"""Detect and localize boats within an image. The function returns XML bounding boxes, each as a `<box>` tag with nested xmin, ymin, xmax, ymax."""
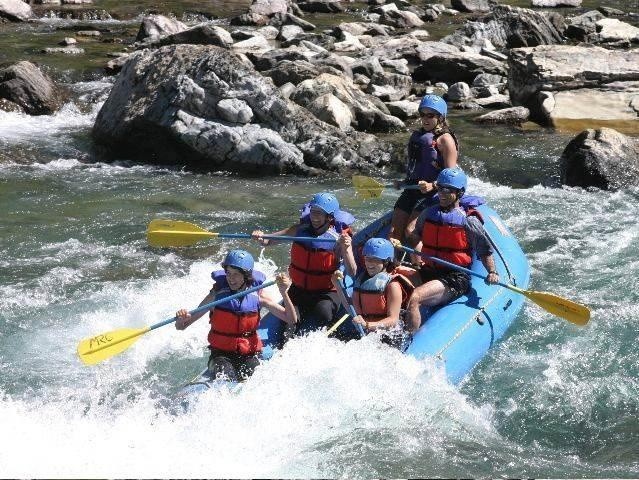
<box><xmin>170</xmin><ymin>193</ymin><xmax>531</xmax><ymax>415</ymax></box>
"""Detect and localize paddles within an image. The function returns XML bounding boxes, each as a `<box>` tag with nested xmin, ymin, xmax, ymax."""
<box><xmin>393</xmin><ymin>243</ymin><xmax>590</xmax><ymax>326</ymax></box>
<box><xmin>353</xmin><ymin>175</ymin><xmax>422</xmax><ymax>200</ymax></box>
<box><xmin>77</xmin><ymin>277</ymin><xmax>277</xmax><ymax>367</ymax></box>
<box><xmin>147</xmin><ymin>219</ymin><xmax>339</xmax><ymax>248</ymax></box>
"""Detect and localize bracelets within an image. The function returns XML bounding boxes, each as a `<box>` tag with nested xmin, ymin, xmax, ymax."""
<box><xmin>432</xmin><ymin>180</ymin><xmax>436</xmax><ymax>191</ymax></box>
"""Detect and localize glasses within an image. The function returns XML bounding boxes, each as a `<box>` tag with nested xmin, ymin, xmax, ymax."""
<box><xmin>437</xmin><ymin>185</ymin><xmax>455</xmax><ymax>194</ymax></box>
<box><xmin>420</xmin><ymin>111</ymin><xmax>440</xmax><ymax>118</ymax></box>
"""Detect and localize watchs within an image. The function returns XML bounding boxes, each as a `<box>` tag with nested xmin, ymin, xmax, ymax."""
<box><xmin>489</xmin><ymin>270</ymin><xmax>499</xmax><ymax>275</ymax></box>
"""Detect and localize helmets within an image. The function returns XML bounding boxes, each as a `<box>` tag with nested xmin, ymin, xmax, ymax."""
<box><xmin>362</xmin><ymin>236</ymin><xmax>395</xmax><ymax>260</ymax></box>
<box><xmin>309</xmin><ymin>192</ymin><xmax>340</xmax><ymax>218</ymax></box>
<box><xmin>224</xmin><ymin>249</ymin><xmax>254</xmax><ymax>273</ymax></box>
<box><xmin>418</xmin><ymin>94</ymin><xmax>448</xmax><ymax>117</ymax></box>
<box><xmin>436</xmin><ymin>167</ymin><xmax>467</xmax><ymax>191</ymax></box>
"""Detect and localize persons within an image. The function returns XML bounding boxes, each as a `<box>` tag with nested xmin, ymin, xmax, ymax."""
<box><xmin>388</xmin><ymin>94</ymin><xmax>459</xmax><ymax>268</ymax></box>
<box><xmin>390</xmin><ymin>167</ymin><xmax>499</xmax><ymax>336</ymax></box>
<box><xmin>330</xmin><ymin>238</ymin><xmax>416</xmax><ymax>354</ymax></box>
<box><xmin>252</xmin><ymin>192</ymin><xmax>357</xmax><ymax>348</ymax></box>
<box><xmin>175</xmin><ymin>249</ymin><xmax>298</xmax><ymax>383</ymax></box>
